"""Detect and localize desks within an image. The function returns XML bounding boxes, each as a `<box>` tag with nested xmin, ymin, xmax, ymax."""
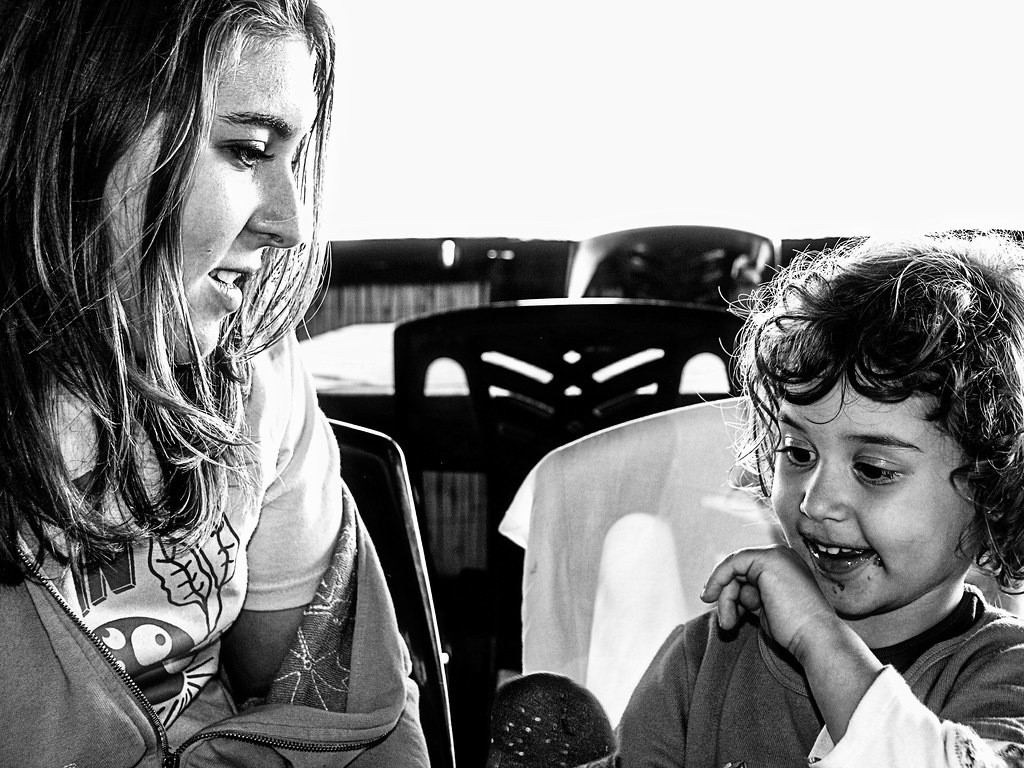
<box><xmin>299</xmin><ymin>322</ymin><xmax>752</xmax><ymax>474</ymax></box>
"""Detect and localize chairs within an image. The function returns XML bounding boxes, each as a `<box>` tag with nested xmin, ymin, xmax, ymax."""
<box><xmin>294</xmin><ymin>227</ymin><xmax>869</xmax><ymax>768</ymax></box>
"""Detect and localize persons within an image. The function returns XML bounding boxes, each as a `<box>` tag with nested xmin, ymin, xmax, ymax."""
<box><xmin>2</xmin><ymin>0</ymin><xmax>435</xmax><ymax>768</ymax></box>
<box><xmin>614</xmin><ymin>227</ymin><xmax>1024</xmax><ymax>768</ymax></box>
